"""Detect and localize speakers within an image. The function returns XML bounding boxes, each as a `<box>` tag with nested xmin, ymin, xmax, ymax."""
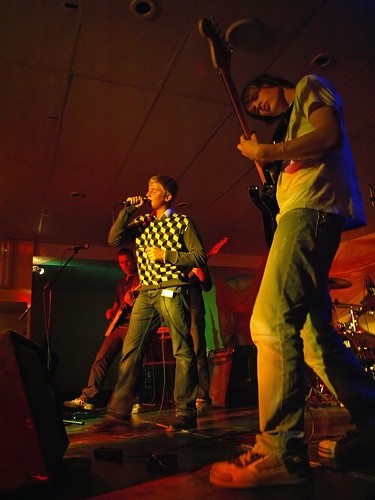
<box><xmin>207</xmin><ymin>345</ymin><xmax>259</xmax><ymax>409</ymax></box>
<box><xmin>0</xmin><ymin>330</ymin><xmax>70</xmax><ymax>500</ymax></box>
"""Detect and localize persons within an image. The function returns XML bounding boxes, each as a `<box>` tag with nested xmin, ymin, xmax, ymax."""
<box><xmin>60</xmin><ymin>175</ymin><xmax>212</xmax><ymax>432</ymax></box>
<box><xmin>208</xmin><ymin>74</ymin><xmax>375</xmax><ymax>490</ymax></box>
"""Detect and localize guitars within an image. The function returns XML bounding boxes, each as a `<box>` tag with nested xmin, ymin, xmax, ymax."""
<box><xmin>188</xmin><ymin>237</ymin><xmax>228</xmax><ymax>278</ymax></box>
<box><xmin>104</xmin><ymin>283</ymin><xmax>142</xmax><ymax>337</ymax></box>
<box><xmin>199</xmin><ymin>15</ymin><xmax>279</xmax><ymax>250</ymax></box>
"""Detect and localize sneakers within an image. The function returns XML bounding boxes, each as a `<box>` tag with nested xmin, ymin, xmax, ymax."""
<box><xmin>63</xmin><ymin>398</ymin><xmax>94</xmax><ymax>410</ymax></box>
<box><xmin>318</xmin><ymin>441</ymin><xmax>338</xmax><ymax>459</ymax></box>
<box><xmin>132</xmin><ymin>404</ymin><xmax>144</xmax><ymax>414</ymax></box>
<box><xmin>209</xmin><ymin>444</ymin><xmax>305</xmax><ymax>486</ymax></box>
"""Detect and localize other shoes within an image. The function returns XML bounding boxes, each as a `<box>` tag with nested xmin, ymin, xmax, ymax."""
<box><xmin>88</xmin><ymin>415</ymin><xmax>126</xmax><ymax>433</ymax></box>
<box><xmin>194</xmin><ymin>400</ymin><xmax>213</xmax><ymax>417</ymax></box>
<box><xmin>172</xmin><ymin>410</ymin><xmax>196</xmax><ymax>430</ymax></box>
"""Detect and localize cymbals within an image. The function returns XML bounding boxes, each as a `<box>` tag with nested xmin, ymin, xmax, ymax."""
<box><xmin>327</xmin><ymin>276</ymin><xmax>352</xmax><ymax>290</ymax></box>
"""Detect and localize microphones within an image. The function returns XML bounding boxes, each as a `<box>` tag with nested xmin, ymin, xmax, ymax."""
<box><xmin>120</xmin><ymin>197</ymin><xmax>147</xmax><ymax>205</ymax></box>
<box><xmin>67</xmin><ymin>243</ymin><xmax>89</xmax><ymax>250</ymax></box>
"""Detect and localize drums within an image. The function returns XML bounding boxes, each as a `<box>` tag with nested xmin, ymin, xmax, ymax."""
<box><xmin>339</xmin><ymin>328</ymin><xmax>375</xmax><ymax>377</ymax></box>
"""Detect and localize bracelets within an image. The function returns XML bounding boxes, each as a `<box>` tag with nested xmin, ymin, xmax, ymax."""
<box><xmin>280</xmin><ymin>142</ymin><xmax>288</xmax><ymax>161</ymax></box>
